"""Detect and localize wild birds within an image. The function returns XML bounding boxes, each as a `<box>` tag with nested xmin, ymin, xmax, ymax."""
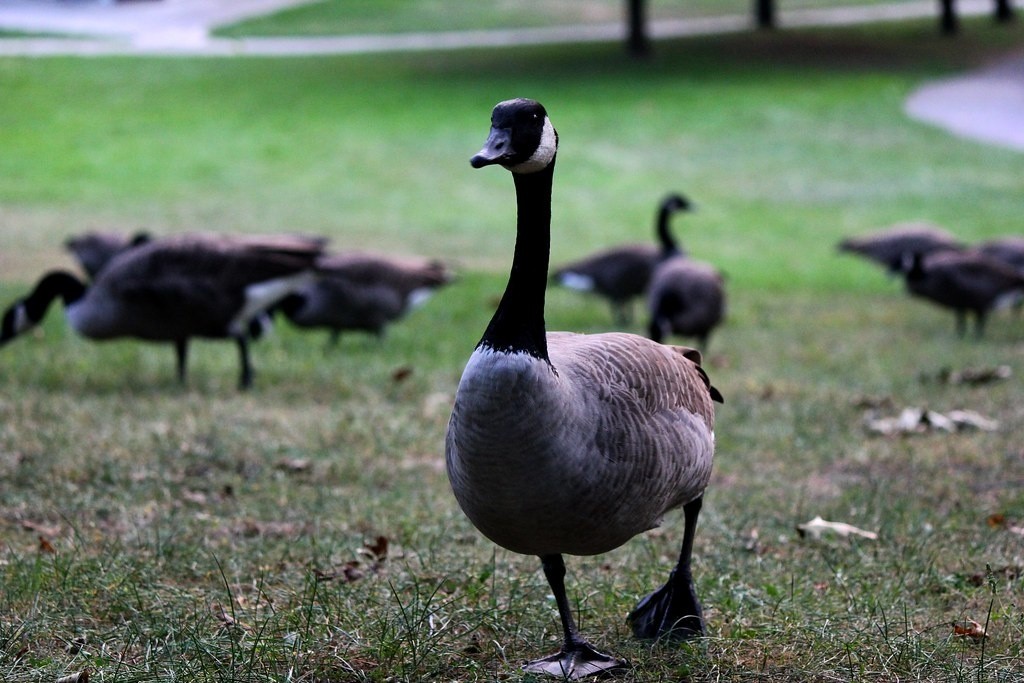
<box><xmin>0</xmin><ymin>225</ymin><xmax>458</xmax><ymax>391</ymax></box>
<box><xmin>445</xmin><ymin>96</ymin><xmax>724</xmax><ymax>683</ymax></box>
<box><xmin>837</xmin><ymin>223</ymin><xmax>1024</xmax><ymax>341</ymax></box>
<box><xmin>549</xmin><ymin>190</ymin><xmax>725</xmax><ymax>356</ymax></box>
